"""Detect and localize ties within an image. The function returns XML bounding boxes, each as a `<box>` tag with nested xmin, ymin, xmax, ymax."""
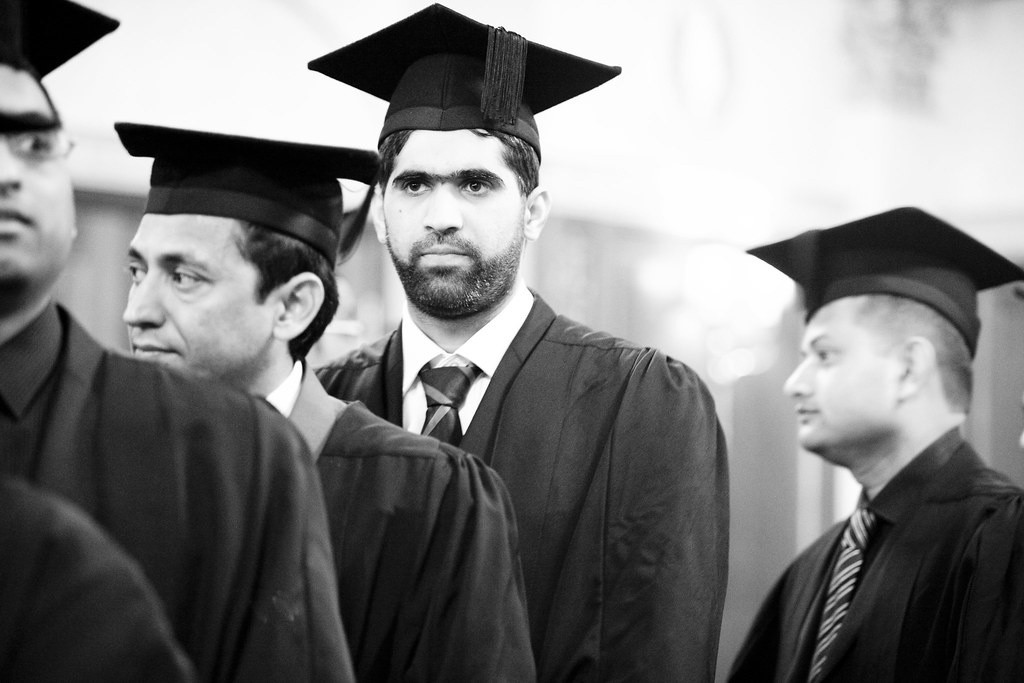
<box><xmin>807</xmin><ymin>510</ymin><xmax>875</xmax><ymax>683</ymax></box>
<box><xmin>415</xmin><ymin>362</ymin><xmax>482</xmax><ymax>447</ymax></box>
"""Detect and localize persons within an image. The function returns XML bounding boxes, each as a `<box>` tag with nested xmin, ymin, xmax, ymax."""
<box><xmin>114</xmin><ymin>122</ymin><xmax>540</xmax><ymax>683</ymax></box>
<box><xmin>307</xmin><ymin>2</ymin><xmax>733</xmax><ymax>683</ymax></box>
<box><xmin>1</xmin><ymin>0</ymin><xmax>356</xmax><ymax>683</ymax></box>
<box><xmin>726</xmin><ymin>202</ymin><xmax>1024</xmax><ymax>682</ymax></box>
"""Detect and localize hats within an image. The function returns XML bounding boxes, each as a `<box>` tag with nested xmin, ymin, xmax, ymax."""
<box><xmin>308</xmin><ymin>3</ymin><xmax>622</xmax><ymax>167</ymax></box>
<box><xmin>745</xmin><ymin>206</ymin><xmax>1024</xmax><ymax>361</ymax></box>
<box><xmin>0</xmin><ymin>0</ymin><xmax>120</xmax><ymax>82</ymax></box>
<box><xmin>114</xmin><ymin>121</ymin><xmax>382</xmax><ymax>271</ymax></box>
